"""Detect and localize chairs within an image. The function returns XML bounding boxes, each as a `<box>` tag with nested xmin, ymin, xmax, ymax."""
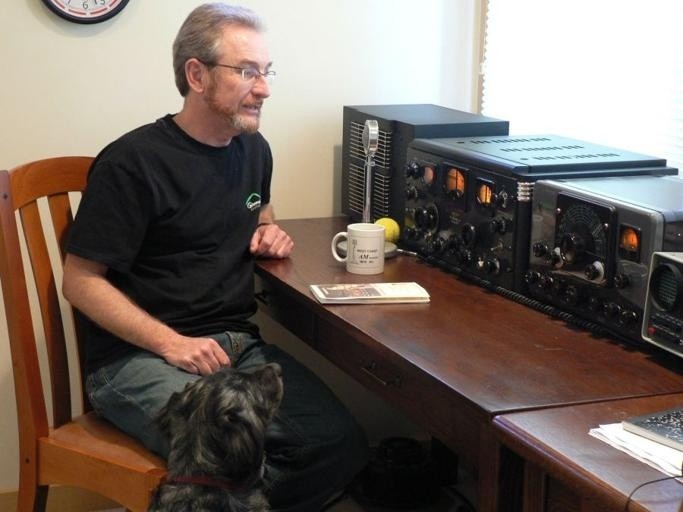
<box><xmin>0</xmin><ymin>156</ymin><xmax>167</xmax><ymax>512</ymax></box>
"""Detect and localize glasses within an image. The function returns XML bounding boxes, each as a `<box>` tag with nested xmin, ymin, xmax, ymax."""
<box><xmin>215</xmin><ymin>61</ymin><xmax>278</xmax><ymax>85</ymax></box>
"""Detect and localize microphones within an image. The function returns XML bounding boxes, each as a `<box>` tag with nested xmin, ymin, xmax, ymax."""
<box><xmin>337</xmin><ymin>119</ymin><xmax>398</xmax><ymax>260</ymax></box>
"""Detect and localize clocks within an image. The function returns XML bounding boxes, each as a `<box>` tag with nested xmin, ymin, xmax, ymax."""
<box><xmin>42</xmin><ymin>1</ymin><xmax>132</xmax><ymax>24</ymax></box>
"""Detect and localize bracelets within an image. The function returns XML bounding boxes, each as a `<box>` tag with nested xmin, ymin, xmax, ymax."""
<box><xmin>254</xmin><ymin>221</ymin><xmax>272</xmax><ymax>228</ymax></box>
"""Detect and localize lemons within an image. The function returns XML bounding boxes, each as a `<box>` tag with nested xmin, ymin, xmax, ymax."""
<box><xmin>374</xmin><ymin>217</ymin><xmax>401</xmax><ymax>242</ymax></box>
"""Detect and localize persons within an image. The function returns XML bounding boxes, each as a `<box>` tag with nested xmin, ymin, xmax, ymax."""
<box><xmin>55</xmin><ymin>1</ymin><xmax>371</xmax><ymax>512</ymax></box>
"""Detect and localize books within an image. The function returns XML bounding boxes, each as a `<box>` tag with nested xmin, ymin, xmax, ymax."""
<box><xmin>308</xmin><ymin>280</ymin><xmax>433</xmax><ymax>306</ymax></box>
<box><xmin>588</xmin><ymin>408</ymin><xmax>683</xmax><ymax>485</ymax></box>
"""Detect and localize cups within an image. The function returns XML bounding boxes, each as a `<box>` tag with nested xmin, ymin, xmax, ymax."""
<box><xmin>330</xmin><ymin>222</ymin><xmax>386</xmax><ymax>276</ymax></box>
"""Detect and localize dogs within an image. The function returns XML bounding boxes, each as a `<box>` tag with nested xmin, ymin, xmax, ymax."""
<box><xmin>146</xmin><ymin>361</ymin><xmax>287</xmax><ymax>512</ymax></box>
